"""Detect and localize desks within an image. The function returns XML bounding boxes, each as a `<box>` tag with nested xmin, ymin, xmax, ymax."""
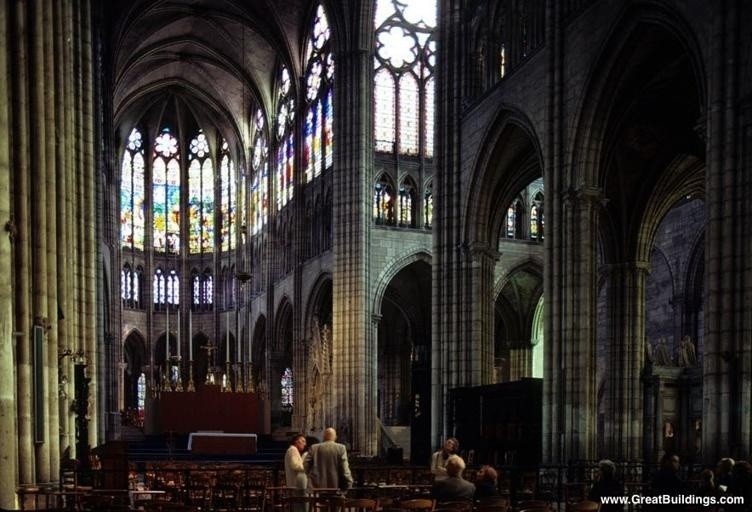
<box><xmin>187</xmin><ymin>432</ymin><xmax>258</xmax><ymax>454</ymax></box>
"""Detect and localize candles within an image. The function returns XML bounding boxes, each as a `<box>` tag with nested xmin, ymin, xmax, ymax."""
<box><xmin>223</xmin><ymin>374</ymin><xmax>226</xmax><ymax>387</ymax></box>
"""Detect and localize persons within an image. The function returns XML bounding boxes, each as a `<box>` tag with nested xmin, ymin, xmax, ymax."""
<box><xmin>308</xmin><ymin>426</ymin><xmax>353</xmax><ymax>511</ymax></box>
<box><xmin>282</xmin><ymin>434</ymin><xmax>310</xmax><ymax>511</ymax></box>
<box><xmin>475</xmin><ymin>465</ymin><xmax>498</xmax><ymax>496</ymax></box>
<box><xmin>430</xmin><ymin>437</ymin><xmax>460</xmax><ymax>482</ymax></box>
<box><xmin>429</xmin><ymin>454</ymin><xmax>475</xmax><ymax>502</ymax></box>
<box><xmin>649</xmin><ymin>452</ymin><xmax>752</xmax><ymax>512</ymax></box>
<box><xmin>586</xmin><ymin>458</ymin><xmax>623</xmax><ymax>512</ymax></box>
